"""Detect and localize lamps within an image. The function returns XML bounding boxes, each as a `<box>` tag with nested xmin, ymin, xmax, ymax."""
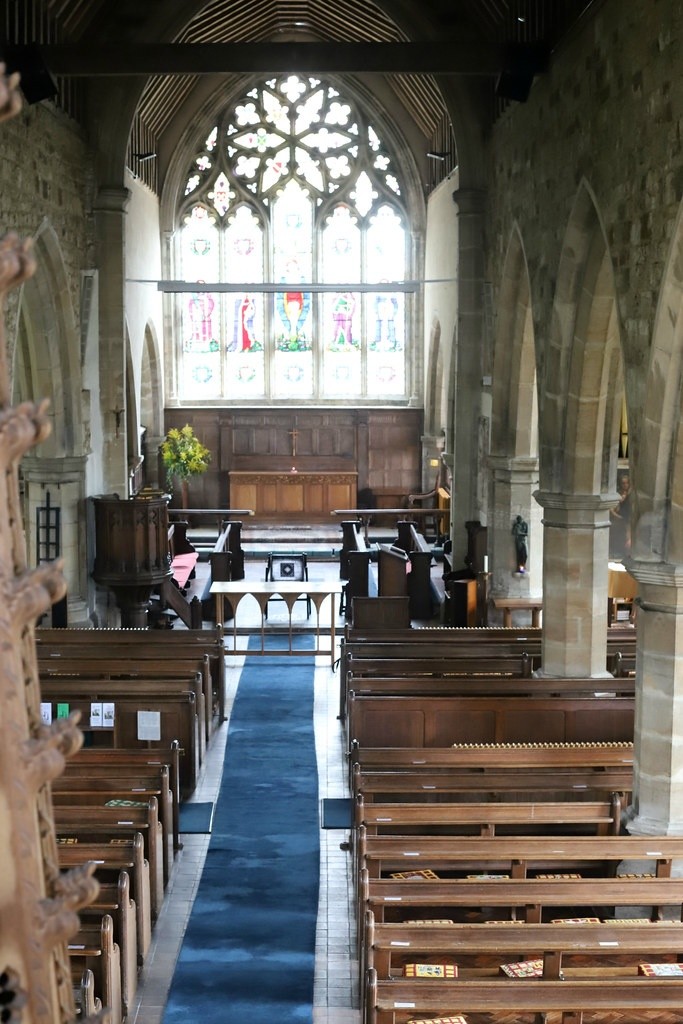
<box><xmin>132</xmin><ymin>153</ymin><xmax>157</xmax><ymax>162</ymax></box>
<box><xmin>427</xmin><ymin>152</ymin><xmax>455</xmax><ymax>160</ymax></box>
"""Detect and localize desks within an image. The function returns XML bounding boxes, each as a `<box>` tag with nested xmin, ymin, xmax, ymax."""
<box><xmin>371</xmin><ymin>489</ymin><xmax>410</xmax><ymax>526</ymax></box>
<box><xmin>492</xmin><ymin>598</ymin><xmax>542</xmax><ymax>628</ymax></box>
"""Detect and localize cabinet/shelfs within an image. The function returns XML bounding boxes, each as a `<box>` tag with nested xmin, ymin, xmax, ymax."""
<box><xmin>227</xmin><ymin>470</ymin><xmax>357</xmax><ymax>523</ymax></box>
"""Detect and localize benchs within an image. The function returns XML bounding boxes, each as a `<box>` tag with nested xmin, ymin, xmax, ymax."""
<box><xmin>33</xmin><ymin>622</ymin><xmax>682</xmax><ymax>1024</ymax></box>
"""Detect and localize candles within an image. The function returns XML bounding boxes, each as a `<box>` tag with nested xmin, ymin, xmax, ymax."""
<box><xmin>484</xmin><ymin>556</ymin><xmax>488</xmax><ymax>573</ymax></box>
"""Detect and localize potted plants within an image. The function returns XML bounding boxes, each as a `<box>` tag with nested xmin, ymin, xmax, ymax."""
<box><xmin>158</xmin><ymin>424</ymin><xmax>212</xmax><ymax>519</ymax></box>
